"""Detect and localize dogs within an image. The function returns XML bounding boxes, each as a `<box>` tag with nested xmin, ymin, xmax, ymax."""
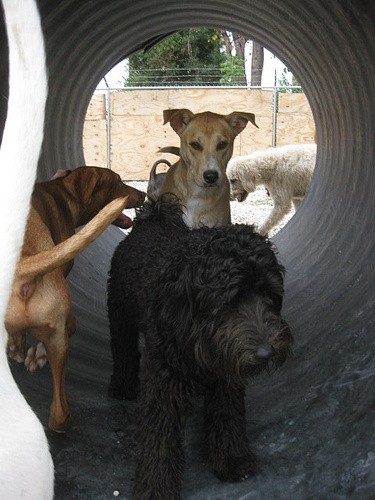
<box><xmin>225</xmin><ymin>143</ymin><xmax>319</xmax><ymax>240</ymax></box>
<box><xmin>106</xmin><ymin>191</ymin><xmax>297</xmax><ymax>500</ymax></box>
<box><xmin>3</xmin><ymin>166</ymin><xmax>147</xmax><ymax>435</ymax></box>
<box><xmin>146</xmin><ymin>108</ymin><xmax>261</xmax><ymax>231</ymax></box>
